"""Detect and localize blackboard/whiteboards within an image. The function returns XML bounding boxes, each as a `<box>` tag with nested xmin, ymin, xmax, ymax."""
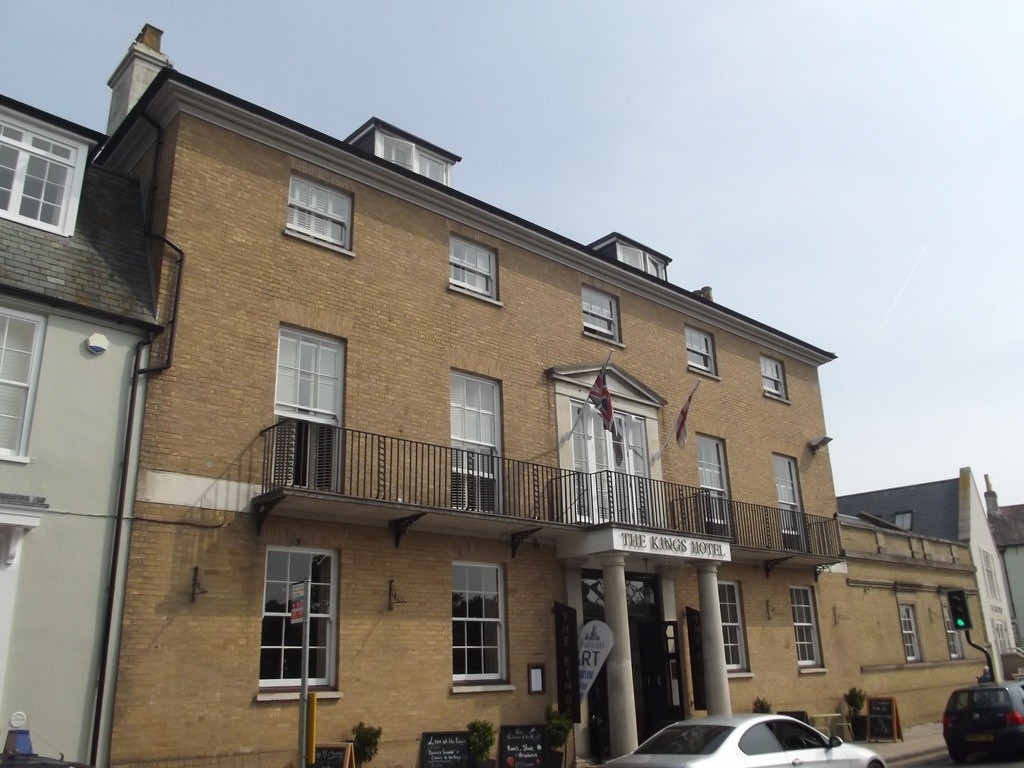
<box><xmin>314</xmin><ymin>742</ymin><xmax>349</xmax><ymax>768</ymax></box>
<box><xmin>500</xmin><ymin>724</ymin><xmax>544</xmax><ymax>768</ymax></box>
<box><xmin>777</xmin><ymin>711</ymin><xmax>805</xmax><ymax>724</ymax></box>
<box><xmin>420</xmin><ymin>730</ymin><xmax>470</xmax><ymax>768</ymax></box>
<box><xmin>866</xmin><ymin>696</ymin><xmax>896</xmax><ymax>740</ymax></box>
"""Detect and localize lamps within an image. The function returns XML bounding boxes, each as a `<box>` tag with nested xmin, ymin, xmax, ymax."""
<box><xmin>809</xmin><ymin>435</ymin><xmax>832</xmax><ymax>449</ymax></box>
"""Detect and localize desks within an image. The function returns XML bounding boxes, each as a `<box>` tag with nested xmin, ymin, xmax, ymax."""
<box><xmin>812</xmin><ymin>713</ymin><xmax>842</xmax><ymax>729</ymax></box>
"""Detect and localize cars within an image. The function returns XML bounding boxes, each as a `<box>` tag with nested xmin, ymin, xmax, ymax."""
<box><xmin>591</xmin><ymin>705</ymin><xmax>891</xmax><ymax>768</ymax></box>
<box><xmin>941</xmin><ymin>679</ymin><xmax>1024</xmax><ymax>762</ymax></box>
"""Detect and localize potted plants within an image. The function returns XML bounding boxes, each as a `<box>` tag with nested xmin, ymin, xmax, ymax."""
<box><xmin>844</xmin><ymin>687</ymin><xmax>866</xmax><ymax>741</ymax></box>
<box><xmin>467</xmin><ymin>719</ymin><xmax>499</xmax><ymax>768</ymax></box>
<box><xmin>539</xmin><ymin>706</ymin><xmax>573</xmax><ymax>768</ymax></box>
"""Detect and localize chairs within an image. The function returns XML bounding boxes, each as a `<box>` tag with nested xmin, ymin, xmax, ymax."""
<box><xmin>833</xmin><ymin>704</ymin><xmax>853</xmax><ymax>743</ymax></box>
<box><xmin>814</xmin><ymin>726</ymin><xmax>831</xmax><ymax>738</ymax></box>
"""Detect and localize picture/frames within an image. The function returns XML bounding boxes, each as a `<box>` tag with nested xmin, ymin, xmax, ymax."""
<box><xmin>528</xmin><ymin>663</ymin><xmax>546</xmax><ymax>695</ymax></box>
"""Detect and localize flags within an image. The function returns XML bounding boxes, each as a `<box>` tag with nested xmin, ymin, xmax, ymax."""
<box><xmin>587</xmin><ymin>364</ymin><xmax>618</xmax><ymax>437</ymax></box>
<box><xmin>675</xmin><ymin>395</ymin><xmax>688</xmax><ymax>450</ymax></box>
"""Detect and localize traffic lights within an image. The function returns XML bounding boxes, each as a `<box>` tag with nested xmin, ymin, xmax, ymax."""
<box><xmin>946</xmin><ymin>589</ymin><xmax>974</xmax><ymax>630</ymax></box>
